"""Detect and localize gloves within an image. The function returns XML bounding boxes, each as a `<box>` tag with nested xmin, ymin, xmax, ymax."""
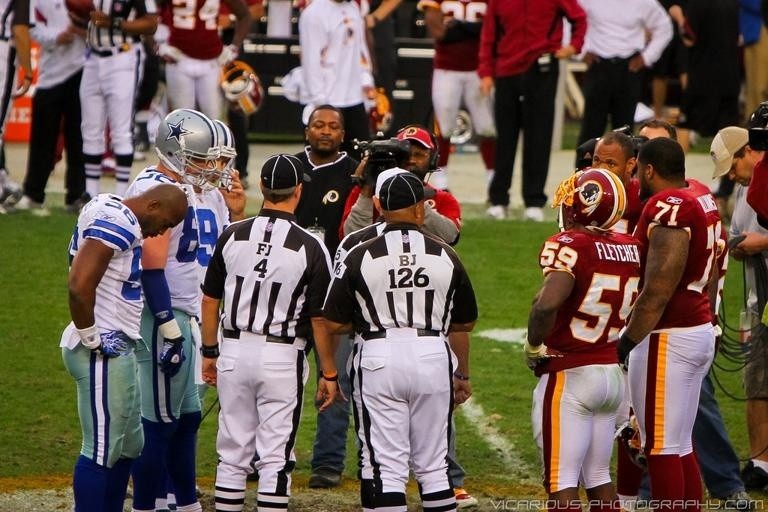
<box><xmin>96</xmin><ymin>330</ymin><xmax>128</xmax><ymax>360</ymax></box>
<box><xmin>217</xmin><ymin>44</ymin><xmax>239</xmax><ymax>62</ymax></box>
<box><xmin>523</xmin><ymin>337</ymin><xmax>548</xmax><ymax>370</ymax></box>
<box><xmin>157</xmin><ymin>335</ymin><xmax>187</xmax><ymax>378</ymax></box>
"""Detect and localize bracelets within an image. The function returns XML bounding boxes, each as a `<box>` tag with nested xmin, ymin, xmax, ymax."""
<box><xmin>322</xmin><ymin>372</ymin><xmax>339</xmax><ymax>384</ymax></box>
<box><xmin>75</xmin><ymin>326</ymin><xmax>102</xmax><ymax>350</ymax></box>
<box><xmin>199</xmin><ymin>343</ymin><xmax>218</xmax><ymax>360</ymax></box>
<box><xmin>453</xmin><ymin>373</ymin><xmax>469</xmax><ymax>382</ymax></box>
<box><xmin>525</xmin><ymin>337</ymin><xmax>542</xmax><ymax>359</ymax></box>
<box><xmin>159</xmin><ymin>319</ymin><xmax>184</xmax><ymax>342</ymax></box>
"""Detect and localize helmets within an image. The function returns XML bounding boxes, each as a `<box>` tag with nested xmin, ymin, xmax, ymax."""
<box><xmin>551</xmin><ymin>167</ymin><xmax>628</xmax><ymax>234</ymax></box>
<box><xmin>366</xmin><ymin>86</ymin><xmax>394</xmax><ymax>134</ymax></box>
<box><xmin>219</xmin><ymin>61</ymin><xmax>263</xmax><ymax>117</ymax></box>
<box><xmin>154</xmin><ymin>109</ymin><xmax>220</xmax><ymax>189</ymax></box>
<box><xmin>210</xmin><ymin>120</ymin><xmax>237</xmax><ymax>191</ymax></box>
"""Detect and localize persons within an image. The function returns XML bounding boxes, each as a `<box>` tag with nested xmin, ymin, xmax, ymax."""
<box><xmin>524</xmin><ymin>167</ymin><xmax>642</xmax><ymax>511</ymax></box>
<box><xmin>123</xmin><ymin>108</ymin><xmax>222</xmax><ymax>512</ymax></box>
<box><xmin>194</xmin><ymin>117</ymin><xmax>246</xmax><ymax>396</ymax></box>
<box><xmin>339</xmin><ymin>124</ymin><xmax>479</xmax><ymax>512</ymax></box>
<box><xmin>595</xmin><ymin>122</ymin><xmax>768</xmax><ymax>512</ymax></box>
<box><xmin>657</xmin><ymin>1</ymin><xmax>764</xmax><ymax>131</ymax></box>
<box><xmin>201</xmin><ymin>151</ymin><xmax>347</xmax><ymax>510</ymax></box>
<box><xmin>59</xmin><ymin>185</ymin><xmax>189</xmax><ymax>511</ymax></box>
<box><xmin>244</xmin><ymin>106</ymin><xmax>359</xmax><ymax>487</ymax></box>
<box><xmin>0</xmin><ymin>0</ymin><xmax>657</xmax><ymax>223</ymax></box>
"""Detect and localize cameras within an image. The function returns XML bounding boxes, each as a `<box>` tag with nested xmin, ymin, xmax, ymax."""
<box><xmin>748</xmin><ymin>102</ymin><xmax>768</xmax><ymax>151</ymax></box>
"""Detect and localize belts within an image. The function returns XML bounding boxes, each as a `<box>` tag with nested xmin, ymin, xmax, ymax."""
<box><xmin>361</xmin><ymin>328</ymin><xmax>439</xmax><ymax>339</ymax></box>
<box><xmin>222</xmin><ymin>330</ymin><xmax>294</xmax><ymax>343</ymax></box>
<box><xmin>91</xmin><ymin>47</ymin><xmax>123</xmax><ymax>58</ymax></box>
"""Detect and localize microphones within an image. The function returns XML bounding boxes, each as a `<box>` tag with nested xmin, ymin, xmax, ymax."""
<box><xmin>410</xmin><ymin>168</ymin><xmax>431</xmax><ymax>186</ymax></box>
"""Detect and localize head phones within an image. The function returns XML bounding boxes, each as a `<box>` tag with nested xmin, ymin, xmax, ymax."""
<box><xmin>394</xmin><ymin>120</ymin><xmax>440</xmax><ymax>169</ymax></box>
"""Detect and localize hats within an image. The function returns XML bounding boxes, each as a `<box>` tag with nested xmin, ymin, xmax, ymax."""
<box><xmin>375</xmin><ymin>166</ymin><xmax>410</xmax><ymax>199</ymax></box>
<box><xmin>745</xmin><ymin>102</ymin><xmax>768</xmax><ymax>131</ymax></box>
<box><xmin>397</xmin><ymin>127</ymin><xmax>434</xmax><ymax>149</ymax></box>
<box><xmin>709</xmin><ymin>125</ymin><xmax>750</xmax><ymax>180</ymax></box>
<box><xmin>379</xmin><ymin>172</ymin><xmax>435</xmax><ymax>210</ymax></box>
<box><xmin>260</xmin><ymin>153</ymin><xmax>311</xmax><ymax>189</ymax></box>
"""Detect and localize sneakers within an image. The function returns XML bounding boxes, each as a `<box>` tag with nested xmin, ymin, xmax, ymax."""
<box><xmin>719</xmin><ymin>491</ymin><xmax>756</xmax><ymax>511</ymax></box>
<box><xmin>523</xmin><ymin>206</ymin><xmax>544</xmax><ymax>223</ymax></box>
<box><xmin>453</xmin><ymin>487</ymin><xmax>479</xmax><ymax>508</ymax></box>
<box><xmin>485</xmin><ymin>204</ymin><xmax>508</xmax><ymax>221</ymax></box>
<box><xmin>307</xmin><ymin>469</ymin><xmax>340</xmax><ymax>488</ymax></box>
<box><xmin>434</xmin><ymin>169</ymin><xmax>449</xmax><ymax>193</ymax></box>
<box><xmin>13</xmin><ymin>195</ymin><xmax>41</xmax><ymax>210</ymax></box>
<box><xmin>741</xmin><ymin>459</ymin><xmax>767</xmax><ymax>493</ymax></box>
